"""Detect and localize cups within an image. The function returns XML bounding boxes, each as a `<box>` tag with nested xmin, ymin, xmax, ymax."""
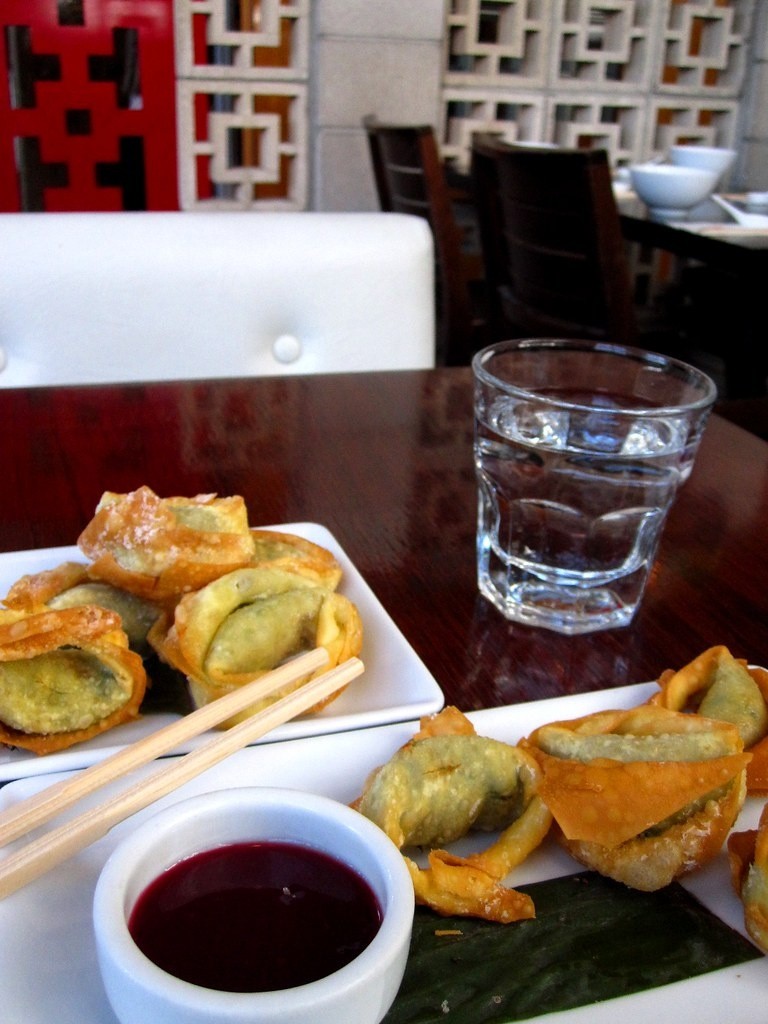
<box><xmin>470</xmin><ymin>338</ymin><xmax>719</xmax><ymax>634</ymax></box>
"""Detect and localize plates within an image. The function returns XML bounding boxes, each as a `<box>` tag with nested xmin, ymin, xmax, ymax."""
<box><xmin>0</xmin><ymin>668</ymin><xmax>768</xmax><ymax>1024</ymax></box>
<box><xmin>0</xmin><ymin>523</ymin><xmax>445</xmax><ymax>787</ymax></box>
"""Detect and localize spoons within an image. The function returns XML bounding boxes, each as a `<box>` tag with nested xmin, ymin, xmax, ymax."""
<box><xmin>710</xmin><ymin>193</ymin><xmax>768</xmax><ymax>229</ymax></box>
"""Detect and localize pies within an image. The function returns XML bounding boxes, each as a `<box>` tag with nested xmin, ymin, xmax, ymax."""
<box><xmin>344</xmin><ymin>642</ymin><xmax>768</xmax><ymax>958</ymax></box>
<box><xmin>0</xmin><ymin>483</ymin><xmax>362</xmax><ymax>754</ymax></box>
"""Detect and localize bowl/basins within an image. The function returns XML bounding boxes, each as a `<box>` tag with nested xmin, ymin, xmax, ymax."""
<box><xmin>90</xmin><ymin>784</ymin><xmax>415</xmax><ymax>1024</ymax></box>
<box><xmin>669</xmin><ymin>146</ymin><xmax>736</xmax><ymax>174</ymax></box>
<box><xmin>628</xmin><ymin>162</ymin><xmax>720</xmax><ymax>218</ymax></box>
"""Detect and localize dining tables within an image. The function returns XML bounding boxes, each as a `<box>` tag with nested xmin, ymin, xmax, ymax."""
<box><xmin>0</xmin><ymin>348</ymin><xmax>768</xmax><ymax>1024</ymax></box>
<box><xmin>620</xmin><ymin>176</ymin><xmax>768</xmax><ymax>311</ymax></box>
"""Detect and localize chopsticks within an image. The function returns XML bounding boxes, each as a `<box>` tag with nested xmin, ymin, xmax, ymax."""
<box><xmin>0</xmin><ymin>648</ymin><xmax>365</xmax><ymax>903</ymax></box>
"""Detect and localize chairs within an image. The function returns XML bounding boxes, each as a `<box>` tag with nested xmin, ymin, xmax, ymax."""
<box><xmin>360</xmin><ymin>112</ymin><xmax>495</xmax><ymax>350</ymax></box>
<box><xmin>0</xmin><ymin>210</ymin><xmax>442</xmax><ymax>390</ymax></box>
<box><xmin>465</xmin><ymin>132</ymin><xmax>670</xmax><ymax>338</ymax></box>
